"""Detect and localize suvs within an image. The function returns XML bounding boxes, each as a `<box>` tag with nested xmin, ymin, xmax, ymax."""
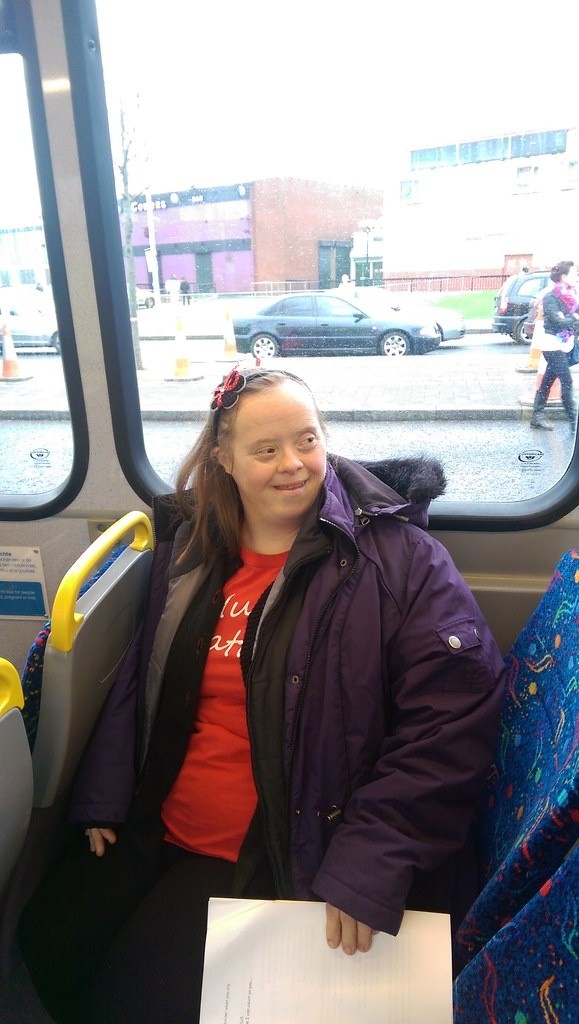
<box><xmin>489</xmin><ymin>267</ymin><xmax>554</xmax><ymax>344</ymax></box>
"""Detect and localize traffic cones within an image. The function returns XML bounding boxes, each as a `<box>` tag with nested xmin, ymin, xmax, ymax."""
<box><xmin>214</xmin><ymin>307</ymin><xmax>248</xmax><ymax>361</ymax></box>
<box><xmin>159</xmin><ymin>312</ymin><xmax>205</xmax><ymax>382</ymax></box>
<box><xmin>0</xmin><ymin>318</ymin><xmax>35</xmax><ymax>383</ymax></box>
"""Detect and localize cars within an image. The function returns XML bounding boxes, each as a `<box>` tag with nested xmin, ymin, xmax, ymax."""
<box><xmin>127</xmin><ymin>285</ymin><xmax>154</xmax><ymax>307</ymax></box>
<box><xmin>0</xmin><ymin>294</ymin><xmax>62</xmax><ymax>355</ymax></box>
<box><xmin>231</xmin><ymin>289</ymin><xmax>468</xmax><ymax>364</ymax></box>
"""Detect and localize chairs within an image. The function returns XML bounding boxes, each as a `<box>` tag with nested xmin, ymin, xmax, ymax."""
<box><xmin>0</xmin><ymin>511</ymin><xmax>155</xmax><ymax>980</ymax></box>
<box><xmin>452</xmin><ymin>550</ymin><xmax>579</xmax><ymax>1024</ymax></box>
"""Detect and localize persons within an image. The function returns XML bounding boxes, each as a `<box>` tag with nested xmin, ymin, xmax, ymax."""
<box><xmin>36</xmin><ymin>283</ymin><xmax>43</xmax><ymax>292</ymax></box>
<box><xmin>180</xmin><ymin>277</ymin><xmax>191</xmax><ymax>305</ymax></box>
<box><xmin>338</xmin><ymin>274</ymin><xmax>353</xmax><ymax>289</ymax></box>
<box><xmin>171</xmin><ymin>273</ymin><xmax>177</xmax><ymax>280</ymax></box>
<box><xmin>530</xmin><ymin>261</ymin><xmax>579</xmax><ymax>432</ymax></box>
<box><xmin>14</xmin><ymin>363</ymin><xmax>508</xmax><ymax>1024</ymax></box>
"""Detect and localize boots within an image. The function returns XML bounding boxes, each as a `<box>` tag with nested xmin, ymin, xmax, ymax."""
<box><xmin>560</xmin><ymin>396</ymin><xmax>576</xmax><ymax>434</ymax></box>
<box><xmin>530</xmin><ymin>389</ymin><xmax>553</xmax><ymax>431</ymax></box>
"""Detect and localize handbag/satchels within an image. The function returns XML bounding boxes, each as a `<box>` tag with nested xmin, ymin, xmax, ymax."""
<box><xmin>567</xmin><ymin>339</ymin><xmax>579</xmax><ymax>367</ymax></box>
